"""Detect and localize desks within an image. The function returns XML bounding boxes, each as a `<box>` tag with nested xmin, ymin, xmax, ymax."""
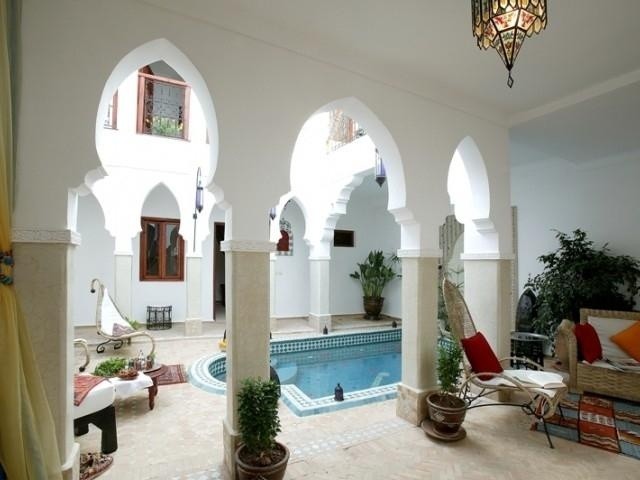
<box><xmin>508</xmin><ymin>331</ymin><xmax>550</xmax><ymax>370</ymax></box>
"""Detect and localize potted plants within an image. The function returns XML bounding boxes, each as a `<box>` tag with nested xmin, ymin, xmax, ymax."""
<box><xmin>420</xmin><ymin>332</ymin><xmax>472</xmax><ymax>441</ymax></box>
<box><xmin>233</xmin><ymin>376</ymin><xmax>291</xmax><ymax>480</ymax></box>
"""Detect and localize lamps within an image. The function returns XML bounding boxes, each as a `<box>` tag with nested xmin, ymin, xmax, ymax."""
<box><xmin>375</xmin><ymin>149</ymin><xmax>385</xmax><ymax>187</ymax></box>
<box><xmin>472</xmin><ymin>0</ymin><xmax>547</xmax><ymax>89</ymax></box>
<box><xmin>193</xmin><ymin>167</ymin><xmax>204</xmax><ymax>219</ymax></box>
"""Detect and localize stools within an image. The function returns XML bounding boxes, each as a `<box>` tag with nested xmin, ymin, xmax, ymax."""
<box><xmin>146</xmin><ymin>303</ymin><xmax>173</xmax><ymax>330</ymax></box>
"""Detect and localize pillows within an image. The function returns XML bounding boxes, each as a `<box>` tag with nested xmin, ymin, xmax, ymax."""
<box><xmin>572</xmin><ymin>315</ymin><xmax>639</xmax><ymax>365</ymax></box>
<box><xmin>460</xmin><ymin>331</ymin><xmax>504</xmax><ymax>382</ymax></box>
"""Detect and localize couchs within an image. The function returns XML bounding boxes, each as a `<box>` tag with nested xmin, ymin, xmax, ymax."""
<box><xmin>555</xmin><ymin>307</ymin><xmax>640</xmax><ymax>404</ymax></box>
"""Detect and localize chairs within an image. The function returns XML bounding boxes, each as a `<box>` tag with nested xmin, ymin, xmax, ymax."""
<box><xmin>90</xmin><ymin>278</ymin><xmax>156</xmax><ymax>357</ymax></box>
<box><xmin>442</xmin><ymin>277</ymin><xmax>569</xmax><ymax>449</ymax></box>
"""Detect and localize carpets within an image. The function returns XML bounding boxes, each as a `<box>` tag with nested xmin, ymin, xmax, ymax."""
<box><xmin>528</xmin><ymin>391</ymin><xmax>640</xmax><ymax>462</ymax></box>
<box><xmin>158</xmin><ymin>365</ymin><xmax>188</xmax><ymax>386</ymax></box>
<box><xmin>349</xmin><ymin>248</ymin><xmax>400</xmax><ymax>321</ymax></box>
